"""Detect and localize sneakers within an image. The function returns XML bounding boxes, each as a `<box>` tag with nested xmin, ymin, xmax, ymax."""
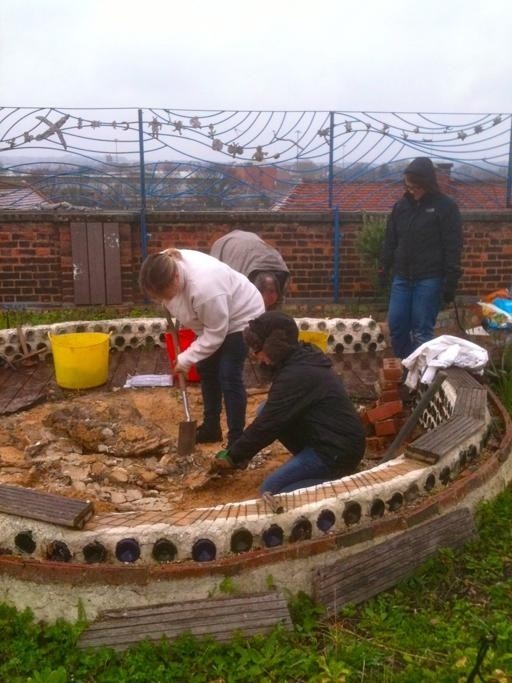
<box><xmin>196</xmin><ymin>423</ymin><xmax>223</xmax><ymax>442</ymax></box>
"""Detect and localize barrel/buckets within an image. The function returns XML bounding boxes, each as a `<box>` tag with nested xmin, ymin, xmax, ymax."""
<box><xmin>46</xmin><ymin>330</ymin><xmax>114</xmax><ymax>389</ymax></box>
<box><xmin>298</xmin><ymin>328</ymin><xmax>330</xmax><ymax>356</ymax></box>
<box><xmin>164</xmin><ymin>329</ymin><xmax>199</xmax><ymax>381</ymax></box>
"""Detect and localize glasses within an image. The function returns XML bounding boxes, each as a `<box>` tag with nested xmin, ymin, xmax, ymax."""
<box><xmin>407</xmin><ymin>186</ymin><xmax>422</xmax><ymax>192</ymax></box>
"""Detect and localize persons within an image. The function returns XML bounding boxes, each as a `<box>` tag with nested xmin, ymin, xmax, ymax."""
<box><xmin>378</xmin><ymin>156</ymin><xmax>463</xmax><ymax>385</ymax></box>
<box><xmin>210</xmin><ymin>229</ymin><xmax>291</xmax><ymax>312</ymax></box>
<box><xmin>213</xmin><ymin>310</ymin><xmax>365</xmax><ymax>495</ymax></box>
<box><xmin>138</xmin><ymin>248</ymin><xmax>267</xmax><ymax>461</ymax></box>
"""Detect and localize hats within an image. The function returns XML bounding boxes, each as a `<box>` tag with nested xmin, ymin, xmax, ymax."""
<box><xmin>242</xmin><ymin>311</ymin><xmax>299</xmax><ymax>361</ymax></box>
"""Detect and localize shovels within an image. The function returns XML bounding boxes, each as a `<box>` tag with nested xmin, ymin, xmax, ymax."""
<box><xmin>164</xmin><ymin>308</ymin><xmax>198</xmax><ymax>456</ymax></box>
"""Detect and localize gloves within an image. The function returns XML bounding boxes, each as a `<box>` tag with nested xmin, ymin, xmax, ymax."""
<box><xmin>442</xmin><ymin>271</ymin><xmax>458</xmax><ymax>302</ymax></box>
<box><xmin>173</xmin><ymin>360</ymin><xmax>189</xmax><ymax>374</ymax></box>
<box><xmin>378</xmin><ymin>264</ymin><xmax>390</xmax><ymax>288</ymax></box>
<box><xmin>211</xmin><ymin>458</ymin><xmax>235</xmax><ymax>473</ymax></box>
